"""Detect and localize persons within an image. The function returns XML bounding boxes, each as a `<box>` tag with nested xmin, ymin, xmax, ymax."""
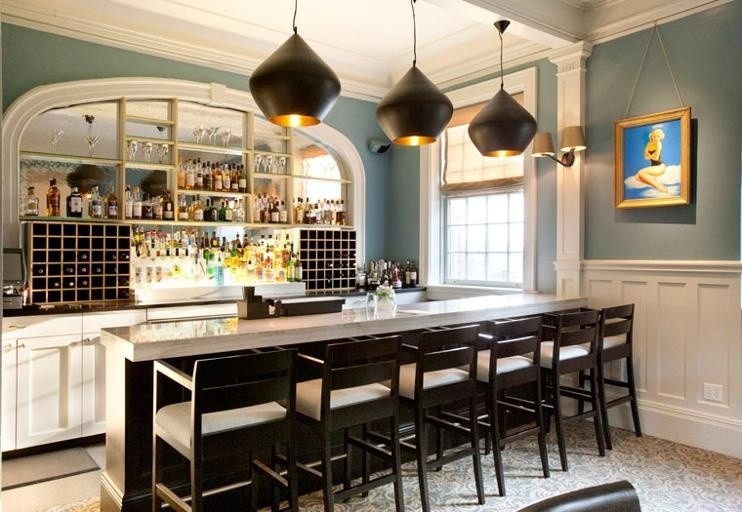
<box><xmin>636</xmin><ymin>129</ymin><xmax>666</xmax><ymax>194</ymax></box>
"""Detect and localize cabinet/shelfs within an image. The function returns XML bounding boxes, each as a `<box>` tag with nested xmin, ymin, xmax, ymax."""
<box><xmin>18</xmin><ymin>97</ymin><xmax>356</xmax><ymax>306</ymax></box>
<box><xmin>0</xmin><ymin>313</ymin><xmax>82</xmax><ymax>454</ymax></box>
<box><xmin>81</xmin><ymin>309</ymin><xmax>146</xmax><ymax>439</ymax></box>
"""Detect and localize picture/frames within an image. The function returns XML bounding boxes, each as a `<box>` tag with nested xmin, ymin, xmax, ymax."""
<box><xmin>613</xmin><ymin>107</ymin><xmax>691</xmax><ymax>209</ymax></box>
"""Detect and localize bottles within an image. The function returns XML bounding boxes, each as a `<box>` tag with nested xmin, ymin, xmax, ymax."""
<box><xmin>37</xmin><ymin>253</ymin><xmax>129</xmax><ymax>288</ymax></box>
<box><xmin>89</xmin><ymin>157</ymin><xmax>348</xmax><ymax>225</ymax></box>
<box><xmin>48</xmin><ymin>178</ymin><xmax>61</xmax><ymax>217</ymax></box>
<box><xmin>326</xmin><ymin>251</ymin><xmax>356</xmax><ymax>278</ymax></box>
<box><xmin>25</xmin><ymin>187</ymin><xmax>39</xmax><ymax>215</ymax></box>
<box><xmin>132</xmin><ymin>225</ymin><xmax>301</xmax><ymax>286</ymax></box>
<box><xmin>67</xmin><ymin>184</ymin><xmax>83</xmax><ymax>217</ymax></box>
<box><xmin>356</xmin><ymin>259</ymin><xmax>418</xmax><ymax>289</ymax></box>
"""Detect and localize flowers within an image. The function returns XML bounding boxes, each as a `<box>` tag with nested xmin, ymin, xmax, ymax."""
<box><xmin>375</xmin><ymin>285</ymin><xmax>396</xmax><ymax>309</ymax></box>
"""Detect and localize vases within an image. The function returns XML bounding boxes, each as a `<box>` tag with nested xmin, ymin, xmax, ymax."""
<box><xmin>376</xmin><ymin>294</ymin><xmax>397</xmax><ymax>311</ymax></box>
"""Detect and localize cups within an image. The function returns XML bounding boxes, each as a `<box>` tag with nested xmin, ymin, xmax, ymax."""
<box><xmin>366</xmin><ymin>293</ymin><xmax>376</xmax><ymax>307</ymax></box>
<box><xmin>367</xmin><ymin>306</ymin><xmax>376</xmax><ymax>321</ymax></box>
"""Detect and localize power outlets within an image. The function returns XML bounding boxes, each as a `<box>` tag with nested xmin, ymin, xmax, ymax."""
<box><xmin>703</xmin><ymin>382</ymin><xmax>723</xmax><ymax>402</ymax></box>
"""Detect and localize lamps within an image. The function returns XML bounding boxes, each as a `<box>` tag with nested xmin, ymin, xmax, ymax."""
<box><xmin>374</xmin><ymin>0</ymin><xmax>455</xmax><ymax>147</ymax></box>
<box><xmin>468</xmin><ymin>20</ymin><xmax>537</xmax><ymax>157</ymax></box>
<box><xmin>531</xmin><ymin>126</ymin><xmax>587</xmax><ymax>167</ymax></box>
<box><xmin>249</xmin><ymin>0</ymin><xmax>342</xmax><ymax>128</ymax></box>
